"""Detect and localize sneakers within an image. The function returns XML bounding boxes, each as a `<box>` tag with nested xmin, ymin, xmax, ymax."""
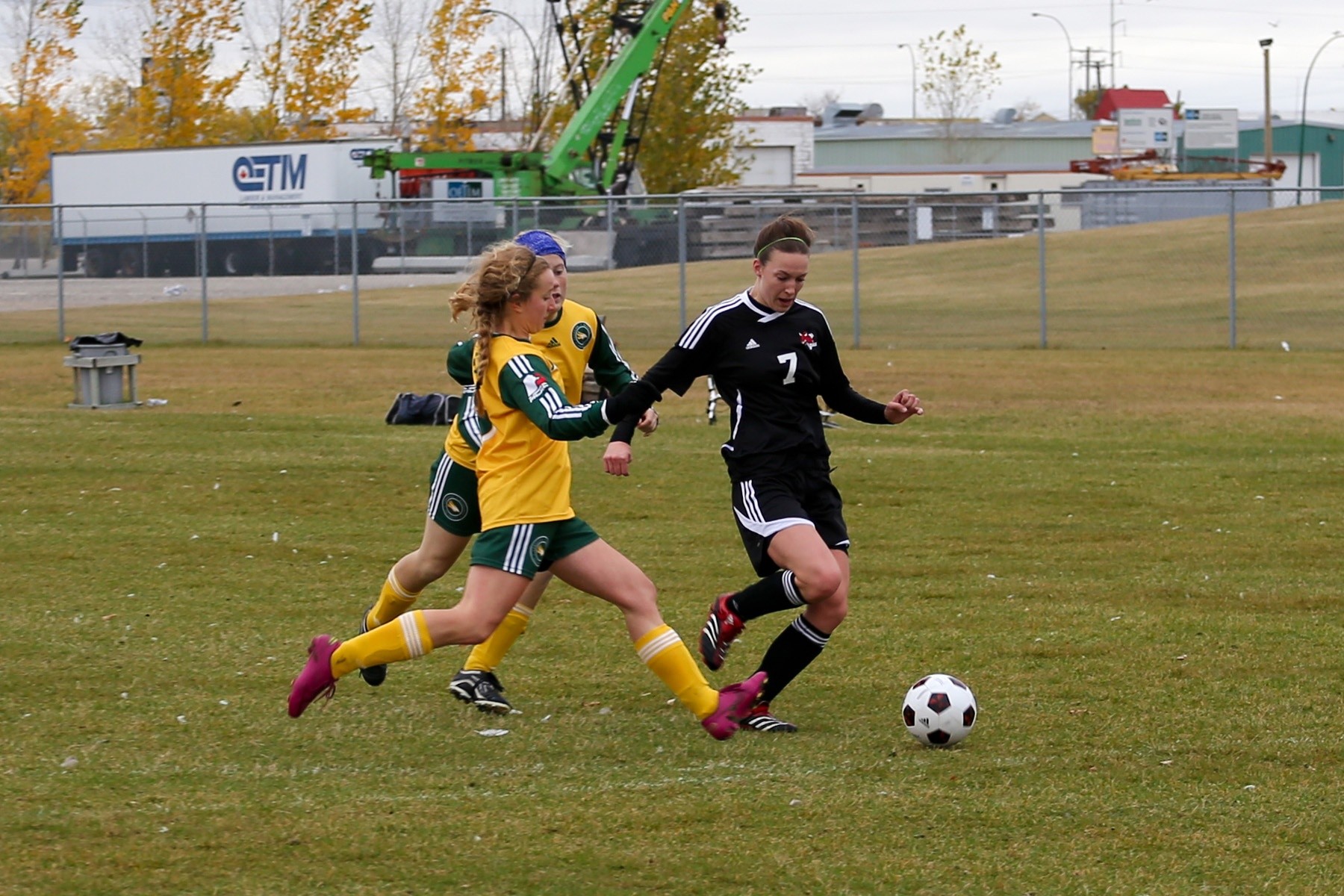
<box><xmin>361</xmin><ymin>607</ymin><xmax>387</xmax><ymax>686</ymax></box>
<box><xmin>450</xmin><ymin>668</ymin><xmax>512</xmax><ymax>716</ymax></box>
<box><xmin>702</xmin><ymin>671</ymin><xmax>768</xmax><ymax>740</ymax></box>
<box><xmin>698</xmin><ymin>592</ymin><xmax>746</xmax><ymax>671</ymax></box>
<box><xmin>287</xmin><ymin>635</ymin><xmax>340</xmax><ymax>718</ymax></box>
<box><xmin>739</xmin><ymin>701</ymin><xmax>797</xmax><ymax>732</ymax></box>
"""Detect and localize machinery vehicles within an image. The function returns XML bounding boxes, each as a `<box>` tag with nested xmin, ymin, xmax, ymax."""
<box><xmin>361</xmin><ymin>0</ymin><xmax>695</xmax><ymax>268</ymax></box>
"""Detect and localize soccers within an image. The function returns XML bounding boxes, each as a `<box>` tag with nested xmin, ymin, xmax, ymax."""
<box><xmin>902</xmin><ymin>672</ymin><xmax>975</xmax><ymax>748</ymax></box>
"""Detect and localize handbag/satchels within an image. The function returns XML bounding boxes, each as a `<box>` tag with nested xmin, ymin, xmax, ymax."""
<box><xmin>385</xmin><ymin>392</ymin><xmax>461</xmax><ymax>426</ymax></box>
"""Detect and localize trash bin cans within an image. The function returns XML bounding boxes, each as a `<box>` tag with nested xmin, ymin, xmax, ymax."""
<box><xmin>61</xmin><ymin>331</ymin><xmax>145</xmax><ymax>412</ymax></box>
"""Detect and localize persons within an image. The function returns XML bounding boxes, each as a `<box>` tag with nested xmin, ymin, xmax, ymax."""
<box><xmin>601</xmin><ymin>219</ymin><xmax>924</xmax><ymax>737</ymax></box>
<box><xmin>286</xmin><ymin>240</ymin><xmax>769</xmax><ymax>742</ymax></box>
<box><xmin>363</xmin><ymin>229</ymin><xmax>662</xmax><ymax>717</ymax></box>
